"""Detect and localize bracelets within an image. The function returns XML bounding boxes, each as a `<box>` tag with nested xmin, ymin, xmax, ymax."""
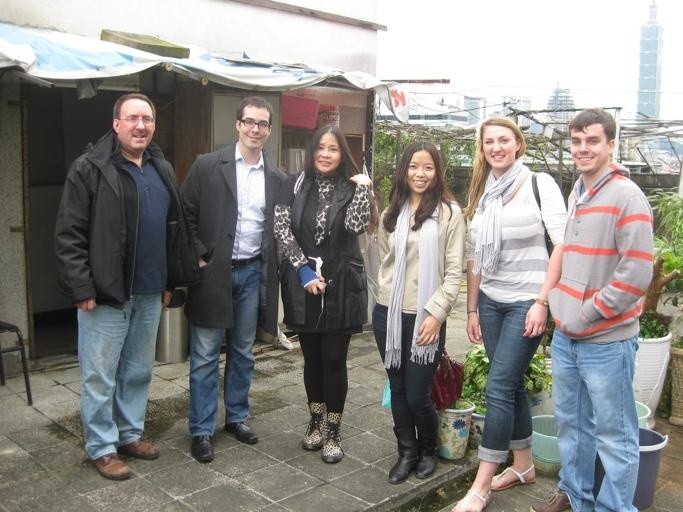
<box><xmin>467</xmin><ymin>310</ymin><xmax>477</xmax><ymax>314</ymax></box>
<box><xmin>535</xmin><ymin>299</ymin><xmax>549</xmax><ymax>307</ymax></box>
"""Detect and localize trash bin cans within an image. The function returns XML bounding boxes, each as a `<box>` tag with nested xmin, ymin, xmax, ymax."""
<box><xmin>155</xmin><ymin>286</ymin><xmax>191</xmax><ymax>365</ymax></box>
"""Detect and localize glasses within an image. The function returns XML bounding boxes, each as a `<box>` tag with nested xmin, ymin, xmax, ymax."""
<box><xmin>239</xmin><ymin>117</ymin><xmax>271</xmax><ymax>129</ymax></box>
<box><xmin>118</xmin><ymin>115</ymin><xmax>155</xmax><ymax>126</ymax></box>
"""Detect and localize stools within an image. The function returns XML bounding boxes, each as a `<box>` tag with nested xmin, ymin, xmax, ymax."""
<box><xmin>0</xmin><ymin>321</ymin><xmax>33</xmax><ymax>405</ymax></box>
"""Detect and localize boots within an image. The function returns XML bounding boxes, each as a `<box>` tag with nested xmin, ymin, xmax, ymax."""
<box><xmin>414</xmin><ymin>429</ymin><xmax>439</xmax><ymax>480</ymax></box>
<box><xmin>321</xmin><ymin>412</ymin><xmax>344</xmax><ymax>465</ymax></box>
<box><xmin>301</xmin><ymin>401</ymin><xmax>323</xmax><ymax>452</ymax></box>
<box><xmin>388</xmin><ymin>426</ymin><xmax>420</xmax><ymax>484</ymax></box>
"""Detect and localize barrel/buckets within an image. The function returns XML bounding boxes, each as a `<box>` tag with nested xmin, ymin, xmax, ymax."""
<box><xmin>594</xmin><ymin>426</ymin><xmax>669</xmax><ymax>510</ymax></box>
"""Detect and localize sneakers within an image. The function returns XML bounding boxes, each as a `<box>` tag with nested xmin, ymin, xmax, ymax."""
<box><xmin>529</xmin><ymin>490</ymin><xmax>571</xmax><ymax>512</ymax></box>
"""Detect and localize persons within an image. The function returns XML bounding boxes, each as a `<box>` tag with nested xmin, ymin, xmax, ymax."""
<box><xmin>272</xmin><ymin>123</ymin><xmax>374</xmax><ymax>464</ymax></box>
<box><xmin>449</xmin><ymin>117</ymin><xmax>567</xmax><ymax>512</ymax></box>
<box><xmin>178</xmin><ymin>96</ymin><xmax>284</xmax><ymax>466</ymax></box>
<box><xmin>526</xmin><ymin>109</ymin><xmax>654</xmax><ymax>512</ymax></box>
<box><xmin>371</xmin><ymin>141</ymin><xmax>467</xmax><ymax>485</ymax></box>
<box><xmin>50</xmin><ymin>93</ymin><xmax>199</xmax><ymax>480</ymax></box>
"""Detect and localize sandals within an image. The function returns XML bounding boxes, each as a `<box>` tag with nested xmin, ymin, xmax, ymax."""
<box><xmin>491</xmin><ymin>462</ymin><xmax>537</xmax><ymax>492</ymax></box>
<box><xmin>450</xmin><ymin>488</ymin><xmax>492</xmax><ymax>512</ymax></box>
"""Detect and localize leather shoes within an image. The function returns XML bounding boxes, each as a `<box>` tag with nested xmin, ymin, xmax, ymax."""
<box><xmin>118</xmin><ymin>438</ymin><xmax>161</xmax><ymax>460</ymax></box>
<box><xmin>223</xmin><ymin>421</ymin><xmax>258</xmax><ymax>445</ymax></box>
<box><xmin>191</xmin><ymin>435</ymin><xmax>215</xmax><ymax>464</ymax></box>
<box><xmin>93</xmin><ymin>453</ymin><xmax>131</xmax><ymax>481</ymax></box>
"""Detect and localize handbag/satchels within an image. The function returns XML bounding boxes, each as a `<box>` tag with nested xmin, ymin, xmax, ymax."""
<box><xmin>431</xmin><ymin>354</ymin><xmax>467</xmax><ymax>411</ymax></box>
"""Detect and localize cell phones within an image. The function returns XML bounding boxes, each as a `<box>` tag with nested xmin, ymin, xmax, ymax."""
<box><xmin>317</xmin><ymin>282</ymin><xmax>327</xmax><ymax>295</ymax></box>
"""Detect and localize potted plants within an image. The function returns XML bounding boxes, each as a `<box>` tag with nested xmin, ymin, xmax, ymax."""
<box><xmin>667</xmin><ymin>335</ymin><xmax>683</xmax><ymax>427</ymax></box>
<box><xmin>460</xmin><ymin>342</ymin><xmax>547</xmax><ymax>450</ymax></box>
<box><xmin>633</xmin><ymin>187</ymin><xmax>683</xmax><ymax>431</ymax></box>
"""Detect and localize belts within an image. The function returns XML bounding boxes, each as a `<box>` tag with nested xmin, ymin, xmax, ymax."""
<box><xmin>231</xmin><ymin>254</ymin><xmax>262</xmax><ymax>269</ymax></box>
